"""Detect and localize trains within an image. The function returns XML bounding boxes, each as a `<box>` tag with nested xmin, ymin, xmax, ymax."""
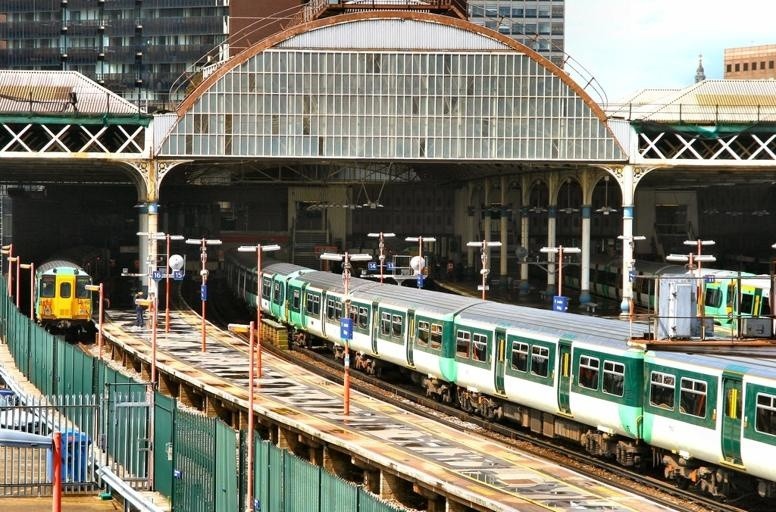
<box><xmin>527</xmin><ymin>244</ymin><xmax>776</xmax><ymax>339</ymax></box>
<box><xmin>32</xmin><ymin>258</ymin><xmax>93</xmax><ymax>338</ymax></box>
<box><xmin>222</xmin><ymin>244</ymin><xmax>776</xmax><ymax>508</ymax></box>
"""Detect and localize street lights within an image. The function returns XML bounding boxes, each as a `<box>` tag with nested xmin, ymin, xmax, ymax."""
<box><xmin>154</xmin><ymin>234</ymin><xmax>188</xmax><ymax>333</ymax></box>
<box><xmin>185</xmin><ymin>238</ymin><xmax>222</xmax><ymax>353</ymax></box>
<box><xmin>238</xmin><ymin>243</ymin><xmax>280</xmax><ymax>378</ymax></box>
<box><xmin>6</xmin><ymin>256</ymin><xmax>20</xmax><ymax>309</ymax></box>
<box><xmin>137</xmin><ymin>296</ymin><xmax>161</xmax><ymax>387</ymax></box>
<box><xmin>19</xmin><ymin>263</ymin><xmax>35</xmax><ymax>322</ymax></box>
<box><xmin>620</xmin><ymin>235</ymin><xmax>649</xmax><ymax>322</ymax></box>
<box><xmin>406</xmin><ymin>236</ymin><xmax>440</xmax><ymax>288</ymax></box>
<box><xmin>319</xmin><ymin>248</ymin><xmax>380</xmax><ymax>422</ymax></box>
<box><xmin>85</xmin><ymin>283</ymin><xmax>103</xmax><ymax>359</ymax></box>
<box><xmin>227</xmin><ymin>321</ymin><xmax>255</xmax><ymax>512</ymax></box>
<box><xmin>542</xmin><ymin>244</ymin><xmax>581</xmax><ymax>311</ymax></box>
<box><xmin>1</xmin><ymin>244</ymin><xmax>13</xmax><ymax>298</ymax></box>
<box><xmin>368</xmin><ymin>232</ymin><xmax>397</xmax><ymax>288</ymax></box>
<box><xmin>684</xmin><ymin>239</ymin><xmax>719</xmax><ymax>341</ymax></box>
<box><xmin>465</xmin><ymin>240</ymin><xmax>508</xmax><ymax>301</ymax></box>
